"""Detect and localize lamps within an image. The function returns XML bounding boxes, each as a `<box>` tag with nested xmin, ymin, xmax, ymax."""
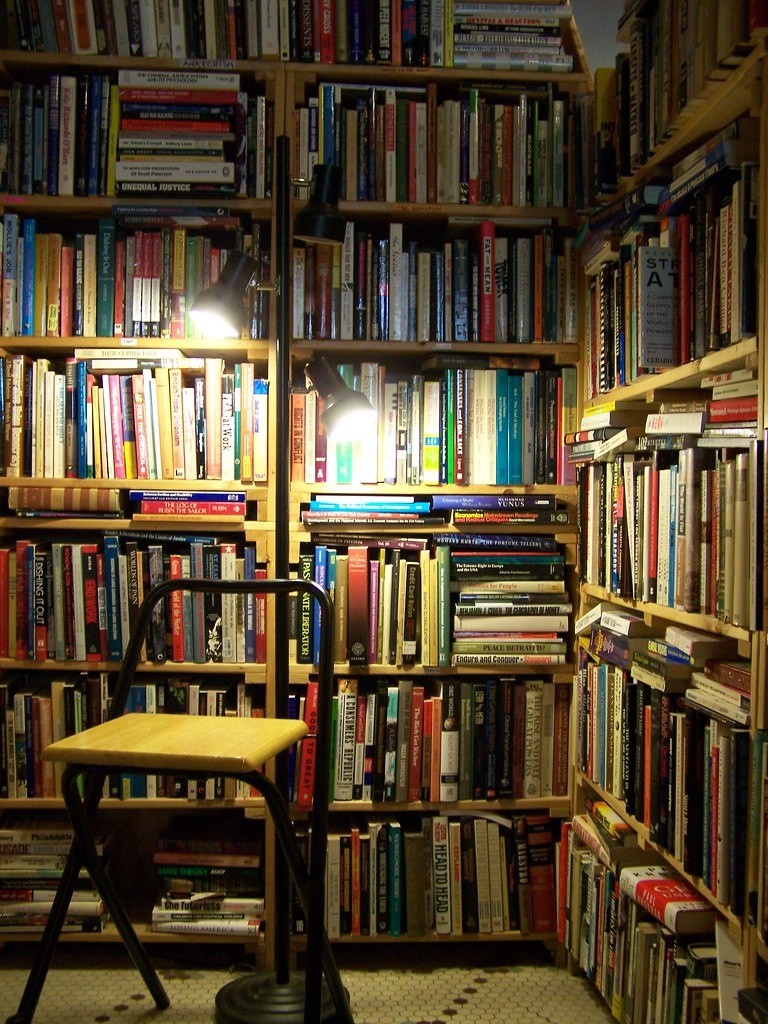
<box><xmin>187</xmin><ymin>137</ymin><xmax>377</xmax><ymax>1023</ymax></box>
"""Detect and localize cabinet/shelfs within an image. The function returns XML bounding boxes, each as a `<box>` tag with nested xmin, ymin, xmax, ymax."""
<box><xmin>1</xmin><ymin>3</ymin><xmax>768</xmax><ymax>1024</ymax></box>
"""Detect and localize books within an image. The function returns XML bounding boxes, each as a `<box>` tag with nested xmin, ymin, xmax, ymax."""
<box><xmin>0</xmin><ymin>348</ymin><xmax>268</xmax><ymax>482</ymax></box>
<box><xmin>0</xmin><ymin>486</ymin><xmax>573</xmax><ymax>664</ymax></box>
<box><xmin>560</xmin><ymin>0</ymin><xmax>768</xmax><ymax>1024</ymax></box>
<box><xmin>291</xmin><ymin>354</ymin><xmax>577</xmax><ymax>487</ymax></box>
<box><xmin>293</xmin><ymin>215</ymin><xmax>578</xmax><ymax>342</ymax></box>
<box><xmin>294</xmin><ymin>82</ymin><xmax>567</xmax><ymax>207</ymax></box>
<box><xmin>0</xmin><ymin>207</ymin><xmax>272</xmax><ymax>338</ymax></box>
<box><xmin>0</xmin><ymin>70</ymin><xmax>274</xmax><ymax>200</ymax></box>
<box><xmin>0</xmin><ymin>0</ymin><xmax>574</xmax><ymax>73</ymax></box>
<box><xmin>0</xmin><ymin>672</ymin><xmax>568</xmax><ymax>802</ymax></box>
<box><xmin>0</xmin><ymin>810</ymin><xmax>557</xmax><ymax>935</ymax></box>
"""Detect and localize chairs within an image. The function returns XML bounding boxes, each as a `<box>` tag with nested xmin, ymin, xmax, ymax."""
<box><xmin>6</xmin><ymin>576</ymin><xmax>359</xmax><ymax>1023</ymax></box>
